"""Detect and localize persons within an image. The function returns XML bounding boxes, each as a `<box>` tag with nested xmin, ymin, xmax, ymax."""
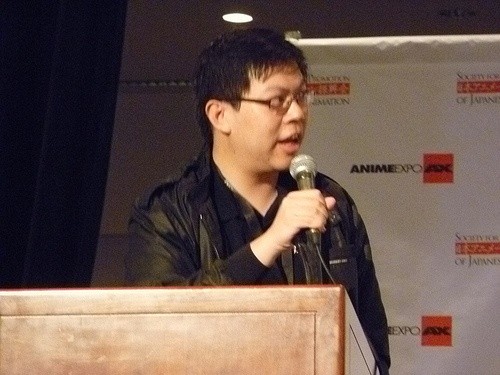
<box><xmin>122</xmin><ymin>25</ymin><xmax>392</xmax><ymax>375</ymax></box>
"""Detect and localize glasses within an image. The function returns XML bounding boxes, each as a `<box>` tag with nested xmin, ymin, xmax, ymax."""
<box><xmin>236</xmin><ymin>89</ymin><xmax>311</xmax><ymax>115</ymax></box>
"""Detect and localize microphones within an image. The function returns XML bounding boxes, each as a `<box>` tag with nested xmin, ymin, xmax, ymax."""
<box><xmin>290</xmin><ymin>154</ymin><xmax>320</xmax><ymax>246</ymax></box>
